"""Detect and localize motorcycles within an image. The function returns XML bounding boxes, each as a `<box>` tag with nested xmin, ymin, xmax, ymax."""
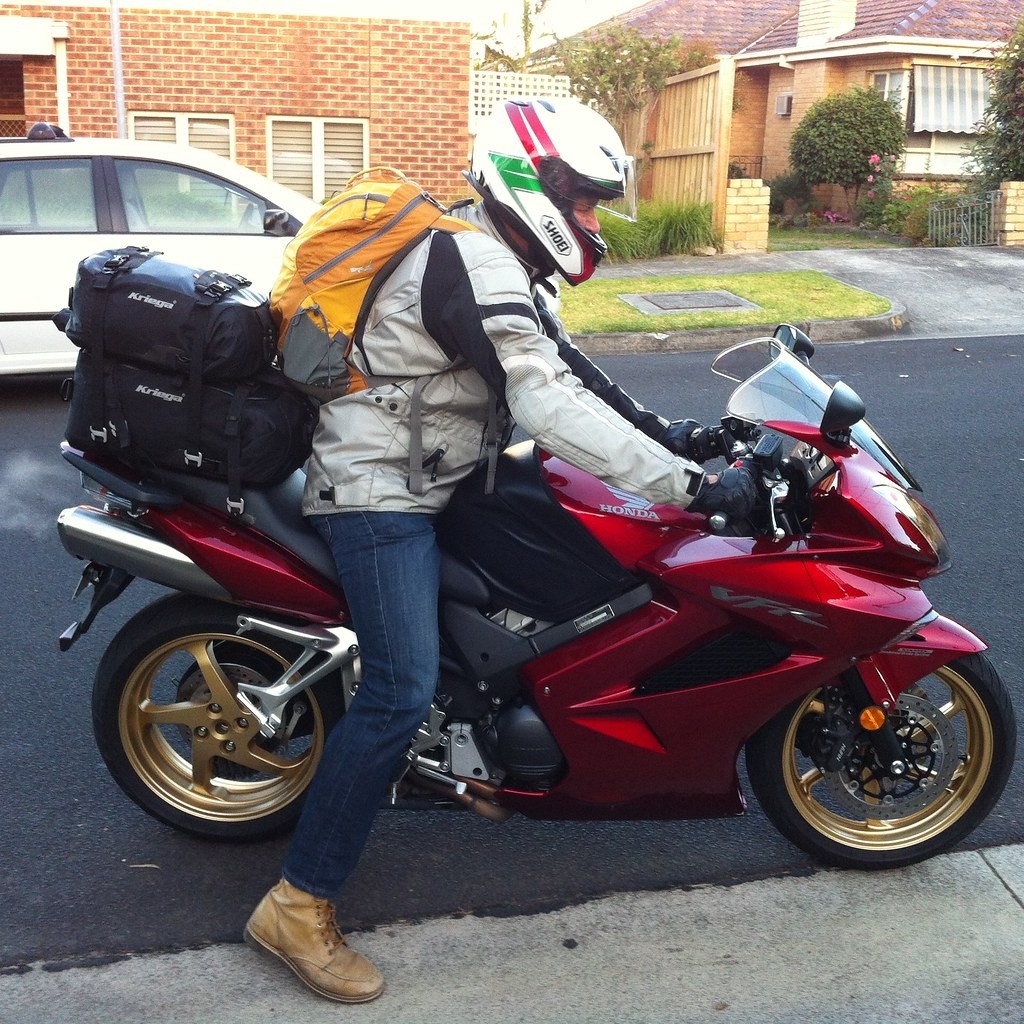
<box><xmin>54</xmin><ymin>324</ymin><xmax>1019</xmax><ymax>871</ymax></box>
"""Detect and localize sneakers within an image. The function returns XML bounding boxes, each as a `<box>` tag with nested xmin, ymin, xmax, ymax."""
<box><xmin>242</xmin><ymin>877</ymin><xmax>384</xmax><ymax>1003</ymax></box>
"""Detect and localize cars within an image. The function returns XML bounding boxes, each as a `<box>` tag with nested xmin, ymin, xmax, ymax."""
<box><xmin>0</xmin><ymin>120</ymin><xmax>328</xmax><ymax>383</ymax></box>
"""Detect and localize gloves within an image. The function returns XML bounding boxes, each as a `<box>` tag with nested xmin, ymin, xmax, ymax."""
<box><xmin>695</xmin><ymin>465</ymin><xmax>759</xmax><ymax>521</ymax></box>
<box><xmin>662</xmin><ymin>419</ymin><xmax>704</xmax><ymax>457</ymax></box>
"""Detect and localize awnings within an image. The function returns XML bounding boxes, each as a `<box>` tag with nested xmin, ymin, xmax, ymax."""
<box><xmin>912</xmin><ymin>66</ymin><xmax>1006</xmax><ymax>136</ymax></box>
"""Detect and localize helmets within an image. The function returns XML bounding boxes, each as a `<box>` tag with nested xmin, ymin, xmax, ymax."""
<box><xmin>470</xmin><ymin>94</ymin><xmax>626</xmax><ymax>287</ymax></box>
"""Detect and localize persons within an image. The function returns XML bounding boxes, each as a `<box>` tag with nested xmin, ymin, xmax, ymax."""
<box><xmin>242</xmin><ymin>98</ymin><xmax>757</xmax><ymax>1004</ymax></box>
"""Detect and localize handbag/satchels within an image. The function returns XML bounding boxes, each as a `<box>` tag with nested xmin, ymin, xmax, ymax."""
<box><xmin>62</xmin><ymin>247</ymin><xmax>317</xmax><ymax>486</ymax></box>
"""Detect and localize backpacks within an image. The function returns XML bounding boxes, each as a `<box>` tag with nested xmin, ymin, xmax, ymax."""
<box><xmin>266</xmin><ymin>165</ymin><xmax>481</xmax><ymax>402</ymax></box>
